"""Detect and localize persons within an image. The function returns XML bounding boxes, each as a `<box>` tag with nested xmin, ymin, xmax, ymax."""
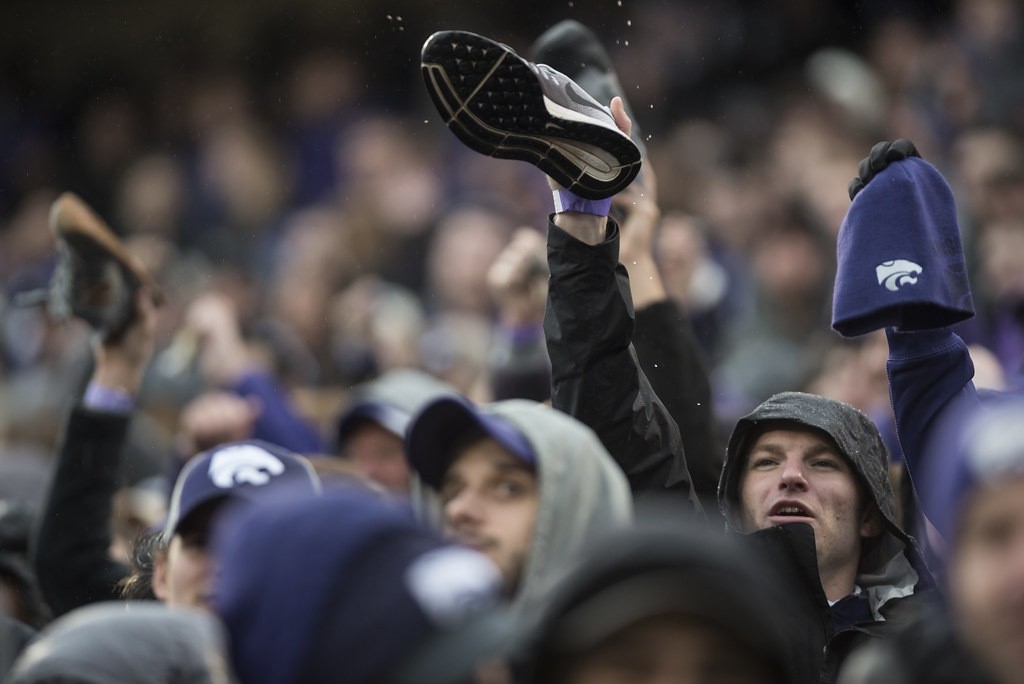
<box><xmin>0</xmin><ymin>0</ymin><xmax>1024</xmax><ymax>684</ymax></box>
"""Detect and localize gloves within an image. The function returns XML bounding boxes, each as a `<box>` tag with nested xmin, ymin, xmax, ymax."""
<box><xmin>847</xmin><ymin>139</ymin><xmax>923</xmax><ymax>200</ymax></box>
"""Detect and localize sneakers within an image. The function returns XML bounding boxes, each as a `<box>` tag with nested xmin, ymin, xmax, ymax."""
<box><xmin>422</xmin><ymin>30</ymin><xmax>642</xmax><ymax>199</ymax></box>
<box><xmin>532</xmin><ymin>19</ymin><xmax>641</xmax><ymax>156</ymax></box>
<box><xmin>49</xmin><ymin>194</ymin><xmax>165</xmax><ymax>344</ymax></box>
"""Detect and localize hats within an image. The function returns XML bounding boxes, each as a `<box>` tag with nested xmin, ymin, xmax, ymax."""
<box><xmin>834</xmin><ymin>160</ymin><xmax>977</xmax><ymax>332</ymax></box>
<box><xmin>334</xmin><ymin>404</ymin><xmax>408</xmax><ymax>445</ymax></box>
<box><xmin>404</xmin><ymin>394</ymin><xmax>538</xmax><ymax>486</ymax></box>
<box><xmin>160</xmin><ymin>441</ymin><xmax>322</xmax><ymax>546</ymax></box>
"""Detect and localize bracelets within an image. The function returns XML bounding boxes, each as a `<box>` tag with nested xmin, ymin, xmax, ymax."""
<box><xmin>77</xmin><ymin>381</ymin><xmax>138</xmax><ymax>414</ymax></box>
<box><xmin>552</xmin><ymin>189</ymin><xmax>611</xmax><ymax>217</ymax></box>
<box><xmin>500</xmin><ymin>324</ymin><xmax>541</xmax><ymax>346</ymax></box>
